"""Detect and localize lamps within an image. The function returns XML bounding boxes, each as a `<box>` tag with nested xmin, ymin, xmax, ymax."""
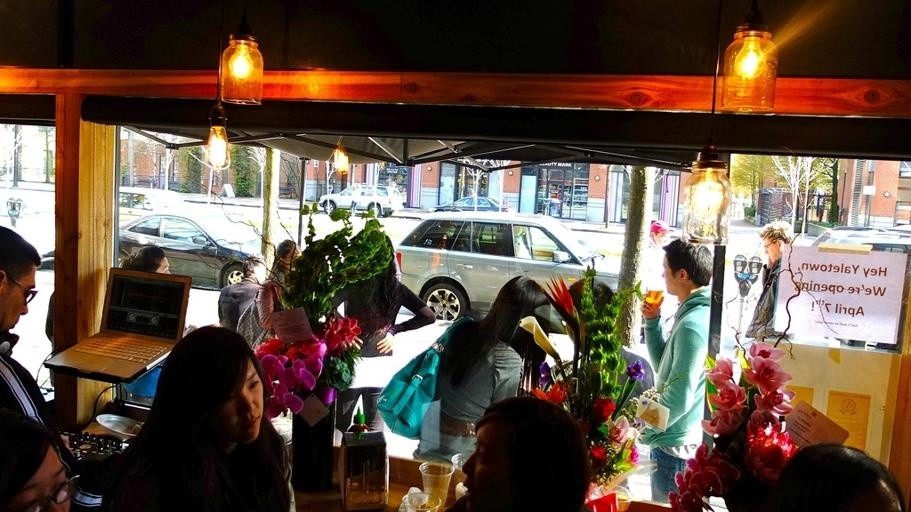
<box><xmin>681</xmin><ymin>0</ymin><xmax>778</xmax><ymax>246</ymax></box>
<box><xmin>205</xmin><ymin>0</ymin><xmax>264</xmax><ymax>170</ymax></box>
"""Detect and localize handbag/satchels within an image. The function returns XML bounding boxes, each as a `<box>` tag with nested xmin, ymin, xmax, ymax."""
<box><xmin>377</xmin><ymin>340</ymin><xmax>441</xmax><ymax>440</ymax></box>
<box><xmin>237</xmin><ymin>301</ymin><xmax>276</xmax><ymax>354</ymax></box>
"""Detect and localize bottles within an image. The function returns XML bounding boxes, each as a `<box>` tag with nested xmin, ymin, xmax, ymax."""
<box><xmin>347</xmin><ymin>408</ymin><xmax>370</xmax><ymax>434</ymax></box>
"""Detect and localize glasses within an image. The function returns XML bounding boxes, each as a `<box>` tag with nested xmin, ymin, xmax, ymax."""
<box><xmin>560</xmin><ymin>318</ymin><xmax>569</xmax><ymax>326</ymax></box>
<box><xmin>8</xmin><ymin>275</ymin><xmax>37</xmax><ymax>304</ymax></box>
<box><xmin>22</xmin><ymin>474</ymin><xmax>80</xmax><ymax>512</ymax></box>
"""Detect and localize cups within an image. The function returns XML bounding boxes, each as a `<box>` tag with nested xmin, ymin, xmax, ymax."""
<box><xmin>416</xmin><ymin>458</ymin><xmax>454</xmax><ymax>511</ymax></box>
<box><xmin>451</xmin><ymin>452</ymin><xmax>472</xmax><ymax>499</ymax></box>
<box><xmin>401</xmin><ymin>492</ymin><xmax>441</xmax><ymax>511</ymax></box>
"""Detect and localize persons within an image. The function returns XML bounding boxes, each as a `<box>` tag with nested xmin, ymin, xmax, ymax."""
<box><xmin>437</xmin><ymin>223</ymin><xmax>459</xmax><ymax>251</ymax></box>
<box><xmin>255</xmin><ymin>239</ymin><xmax>303</xmax><ymax>339</ymax></box>
<box><xmin>217</xmin><ymin>255</ymin><xmax>269</xmax><ymax>335</ymax></box>
<box><xmin>329</xmin><ymin>234</ymin><xmax>436</xmax><ymax>437</ymax></box>
<box><xmin>411</xmin><ymin>274</ymin><xmax>552</xmax><ymax>471</ymax></box>
<box><xmin>633</xmin><ymin>219</ymin><xmax>676</xmax><ymax>343</ymax></box>
<box><xmin>772</xmin><ymin>440</ymin><xmax>908</xmax><ymax>511</ymax></box>
<box><xmin>440</xmin><ymin>396</ymin><xmax>594</xmax><ymax>511</ymax></box>
<box><xmin>744</xmin><ymin>219</ymin><xmax>796</xmax><ymax>344</ymax></box>
<box><xmin>637</xmin><ymin>236</ymin><xmax>714</xmax><ymax>506</ymax></box>
<box><xmin>0</xmin><ymin>404</ymin><xmax>77</xmax><ymax>511</ymax></box>
<box><xmin>561</xmin><ymin>277</ymin><xmax>656</xmax><ymax>411</ymax></box>
<box><xmin>121</xmin><ymin>246</ymin><xmax>199</xmax><ymax>341</ymax></box>
<box><xmin>66</xmin><ymin>322</ymin><xmax>299</xmax><ymax>512</ymax></box>
<box><xmin>0</xmin><ymin>223</ymin><xmax>82</xmax><ymax>486</ymax></box>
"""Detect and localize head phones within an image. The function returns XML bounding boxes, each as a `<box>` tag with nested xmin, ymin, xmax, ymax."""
<box><xmin>0</xmin><ymin>330</ymin><xmax>19</xmax><ymax>356</ymax></box>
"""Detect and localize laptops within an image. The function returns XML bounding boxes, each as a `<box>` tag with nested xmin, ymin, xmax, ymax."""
<box><xmin>43</xmin><ymin>266</ymin><xmax>193</xmax><ymax>383</ymax></box>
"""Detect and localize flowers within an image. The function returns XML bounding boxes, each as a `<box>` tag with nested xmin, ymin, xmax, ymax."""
<box><xmin>667</xmin><ymin>230</ymin><xmax>798</xmax><ymax>512</ymax></box>
<box><xmin>521</xmin><ymin>258</ymin><xmax>687</xmax><ymax>497</ymax></box>
<box><xmin>188</xmin><ymin>178</ymin><xmax>394</xmax><ymax>421</ymax></box>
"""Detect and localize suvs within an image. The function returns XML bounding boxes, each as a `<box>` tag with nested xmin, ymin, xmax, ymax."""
<box><xmin>320</xmin><ymin>183</ymin><xmax>405</xmax><ymax>218</ymax></box>
<box><xmin>395</xmin><ymin>214</ymin><xmax>673</xmax><ymax>343</ymax></box>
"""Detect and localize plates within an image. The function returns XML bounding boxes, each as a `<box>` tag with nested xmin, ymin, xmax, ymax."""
<box><xmin>96</xmin><ymin>414</ymin><xmax>137</xmax><ymax>437</ymax></box>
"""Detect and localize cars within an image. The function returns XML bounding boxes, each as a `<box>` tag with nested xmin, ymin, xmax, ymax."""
<box><xmin>119</xmin><ymin>215</ymin><xmax>260</xmax><ymax>290</ymax></box>
<box><xmin>811</xmin><ymin>225</ymin><xmax>910</xmax><ymax>255</ymax></box>
<box><xmin>537</xmin><ymin>192</ymin><xmax>563</xmax><ymax>214</ymax></box>
<box><xmin>431</xmin><ymin>196</ymin><xmax>507</xmax><ymax>213</ymax></box>
